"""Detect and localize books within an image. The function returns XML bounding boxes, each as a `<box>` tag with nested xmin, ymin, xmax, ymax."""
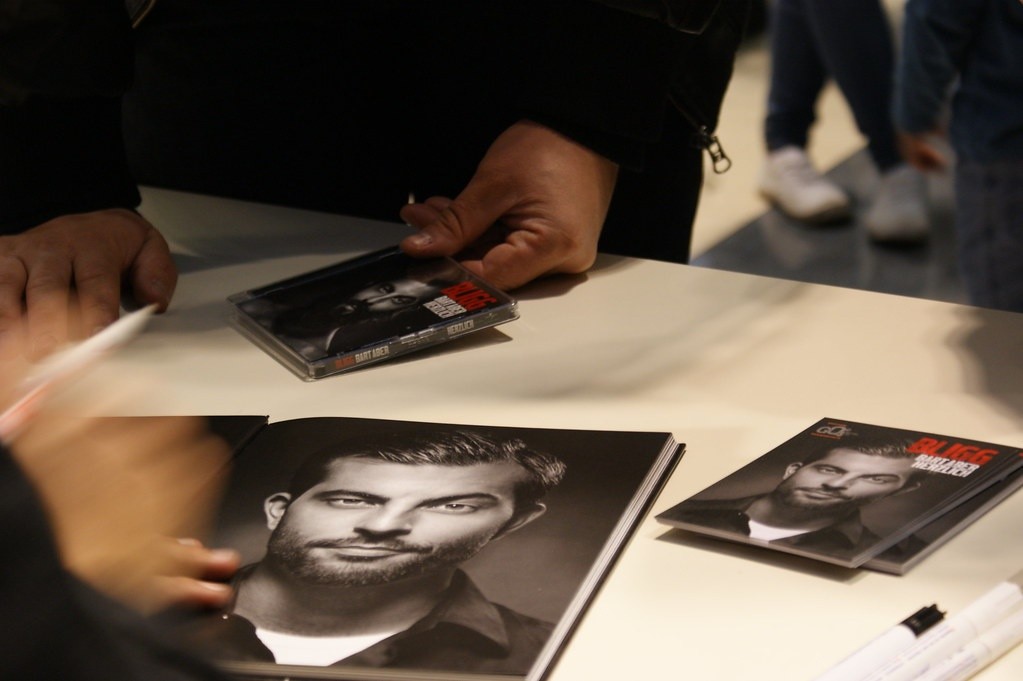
<box><xmin>655</xmin><ymin>417</ymin><xmax>1023</xmax><ymax>578</ymax></box>
<box><xmin>102</xmin><ymin>415</ymin><xmax>686</xmax><ymax>681</ymax></box>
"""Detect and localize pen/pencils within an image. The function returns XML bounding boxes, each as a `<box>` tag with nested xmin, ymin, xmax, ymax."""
<box><xmin>0</xmin><ymin>301</ymin><xmax>160</xmax><ymax>446</ymax></box>
<box><xmin>811</xmin><ymin>580</ymin><xmax>1023</xmax><ymax>681</ymax></box>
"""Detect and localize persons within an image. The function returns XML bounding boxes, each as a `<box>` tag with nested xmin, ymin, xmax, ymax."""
<box><xmin>166</xmin><ymin>428</ymin><xmax>564</xmax><ymax>675</ymax></box>
<box><xmin>1</xmin><ymin>0</ymin><xmax>767</xmax><ymax>681</ymax></box>
<box><xmin>266</xmin><ymin>272</ymin><xmax>446</xmax><ymax>359</ymax></box>
<box><xmin>676</xmin><ymin>434</ymin><xmax>928</xmax><ymax>560</ymax></box>
<box><xmin>761</xmin><ymin>1</ymin><xmax>1023</xmax><ymax>311</ymax></box>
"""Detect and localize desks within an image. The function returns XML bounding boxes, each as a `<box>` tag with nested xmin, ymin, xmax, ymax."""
<box><xmin>0</xmin><ymin>184</ymin><xmax>1023</xmax><ymax>681</ymax></box>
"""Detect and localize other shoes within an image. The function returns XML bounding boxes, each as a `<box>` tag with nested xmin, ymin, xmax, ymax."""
<box><xmin>754</xmin><ymin>147</ymin><xmax>851</xmax><ymax>223</ymax></box>
<box><xmin>865</xmin><ymin>167</ymin><xmax>932</xmax><ymax>242</ymax></box>
